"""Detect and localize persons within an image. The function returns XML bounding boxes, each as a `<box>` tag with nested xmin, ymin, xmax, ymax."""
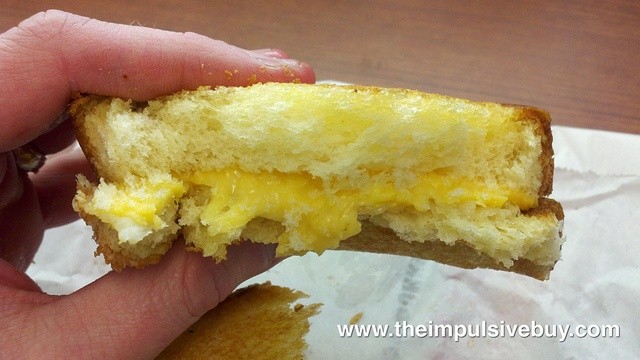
<box><xmin>0</xmin><ymin>8</ymin><xmax>317</xmax><ymax>360</ymax></box>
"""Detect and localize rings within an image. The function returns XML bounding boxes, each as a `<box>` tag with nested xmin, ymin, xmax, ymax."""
<box><xmin>13</xmin><ymin>143</ymin><xmax>47</xmax><ymax>173</ymax></box>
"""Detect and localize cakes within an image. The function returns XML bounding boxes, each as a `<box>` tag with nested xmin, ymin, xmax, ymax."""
<box><xmin>166</xmin><ymin>283</ymin><xmax>324</xmax><ymax>358</ymax></box>
<box><xmin>71</xmin><ymin>83</ymin><xmax>564</xmax><ymax>280</ymax></box>
